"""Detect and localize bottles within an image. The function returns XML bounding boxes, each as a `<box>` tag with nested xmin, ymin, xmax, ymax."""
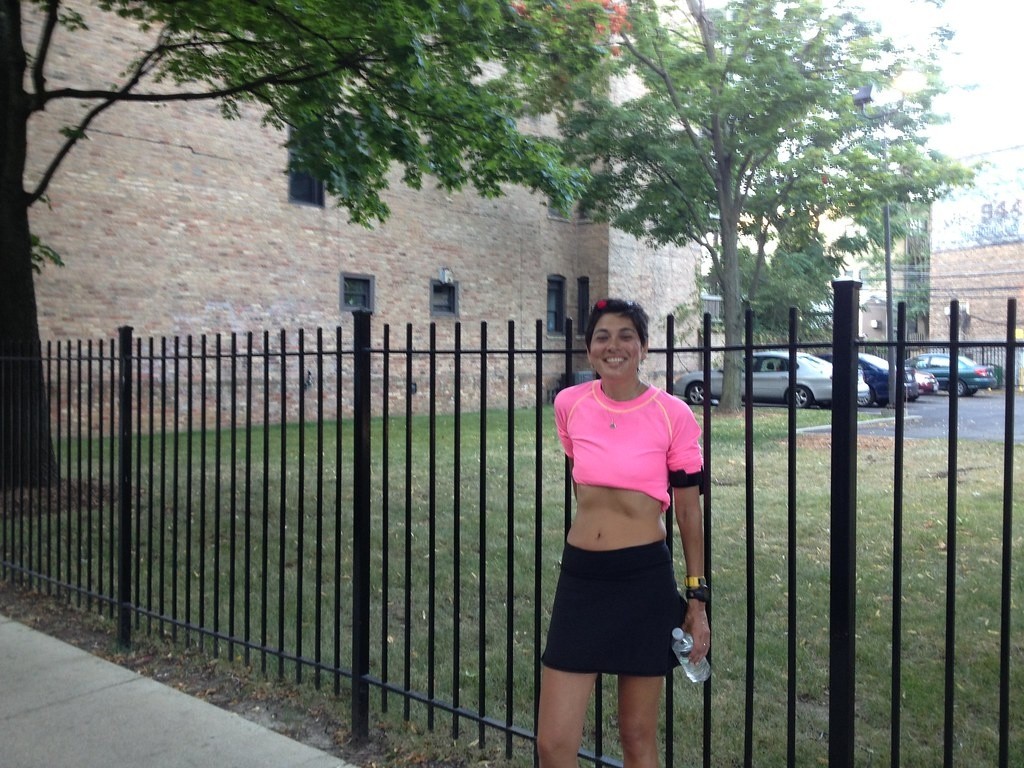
<box><xmin>671</xmin><ymin>626</ymin><xmax>710</xmax><ymax>683</ymax></box>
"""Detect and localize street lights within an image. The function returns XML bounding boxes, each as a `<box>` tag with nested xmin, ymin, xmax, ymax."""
<box><xmin>852</xmin><ymin>70</ymin><xmax>929</xmax><ymax>402</ymax></box>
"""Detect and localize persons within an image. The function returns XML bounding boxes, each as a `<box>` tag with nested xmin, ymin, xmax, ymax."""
<box><xmin>537</xmin><ymin>300</ymin><xmax>710</xmax><ymax>768</ymax></box>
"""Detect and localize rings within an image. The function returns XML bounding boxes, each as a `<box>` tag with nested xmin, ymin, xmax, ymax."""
<box><xmin>704</xmin><ymin>643</ymin><xmax>707</xmax><ymax>646</ymax></box>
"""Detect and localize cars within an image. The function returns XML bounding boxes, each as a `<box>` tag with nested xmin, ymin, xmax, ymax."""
<box><xmin>915</xmin><ymin>370</ymin><xmax>940</xmax><ymax>393</ymax></box>
<box><xmin>904</xmin><ymin>354</ymin><xmax>997</xmax><ymax>398</ymax></box>
<box><xmin>779</xmin><ymin>352</ymin><xmax>919</xmax><ymax>408</ymax></box>
<box><xmin>673</xmin><ymin>351</ymin><xmax>871</xmax><ymax>408</ymax></box>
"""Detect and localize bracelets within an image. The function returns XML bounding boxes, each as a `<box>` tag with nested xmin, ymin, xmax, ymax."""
<box><xmin>684</xmin><ymin>576</ymin><xmax>708</xmax><ymax>604</ymax></box>
